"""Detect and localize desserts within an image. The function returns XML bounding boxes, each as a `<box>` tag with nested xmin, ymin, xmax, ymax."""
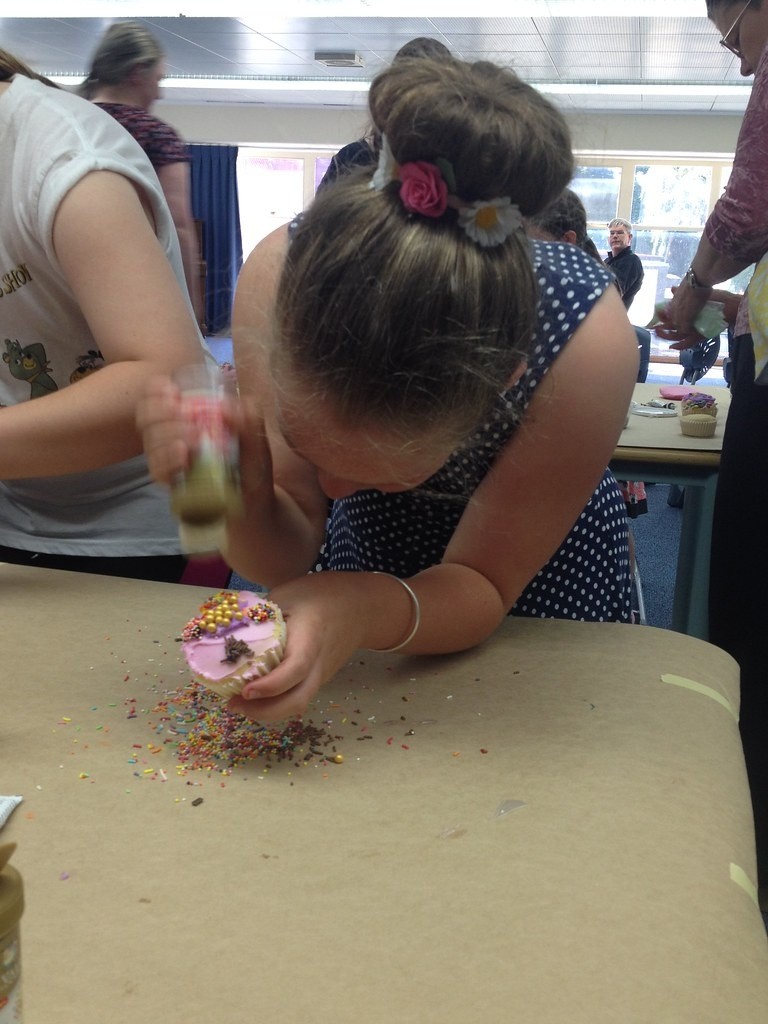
<box><xmin>180</xmin><ymin>590</ymin><xmax>286</xmax><ymax>700</ymax></box>
<box><xmin>683</xmin><ymin>392</ymin><xmax>717</xmax><ymax>414</ymax></box>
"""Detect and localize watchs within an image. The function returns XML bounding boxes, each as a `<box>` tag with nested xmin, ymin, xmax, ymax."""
<box><xmin>686</xmin><ymin>268</ymin><xmax>713</xmax><ymax>295</ymax></box>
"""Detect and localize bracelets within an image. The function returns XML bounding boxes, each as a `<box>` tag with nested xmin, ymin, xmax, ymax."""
<box><xmin>368</xmin><ymin>571</ymin><xmax>419</xmax><ymax>652</ymax></box>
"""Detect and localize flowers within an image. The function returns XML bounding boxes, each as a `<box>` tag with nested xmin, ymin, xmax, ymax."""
<box><xmin>368</xmin><ymin>131</ymin><xmax>398</xmax><ymax>194</ymax></box>
<box><xmin>395</xmin><ymin>161</ymin><xmax>448</xmax><ymax>219</ymax></box>
<box><xmin>458</xmin><ymin>193</ymin><xmax>523</xmax><ymax>250</ymax></box>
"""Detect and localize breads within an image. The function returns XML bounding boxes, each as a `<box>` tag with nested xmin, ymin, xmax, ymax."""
<box><xmin>679</xmin><ymin>414</ymin><xmax>717</xmax><ymax>438</ymax></box>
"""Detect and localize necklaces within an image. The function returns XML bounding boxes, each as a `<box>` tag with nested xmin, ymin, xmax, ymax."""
<box><xmin>138</xmin><ymin>60</ymin><xmax>640</xmax><ymax>719</ymax></box>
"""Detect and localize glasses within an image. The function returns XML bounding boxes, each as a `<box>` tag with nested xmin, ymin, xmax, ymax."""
<box><xmin>720</xmin><ymin>0</ymin><xmax>752</xmax><ymax>58</ymax></box>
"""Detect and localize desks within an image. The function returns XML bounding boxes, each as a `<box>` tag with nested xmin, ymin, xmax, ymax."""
<box><xmin>595</xmin><ymin>377</ymin><xmax>736</xmax><ymax>634</ymax></box>
<box><xmin>0</xmin><ymin>561</ymin><xmax>768</xmax><ymax>1024</ymax></box>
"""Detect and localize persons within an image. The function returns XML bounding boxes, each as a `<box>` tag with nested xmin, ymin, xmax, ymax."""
<box><xmin>317</xmin><ymin>38</ymin><xmax>448</xmax><ymax>187</ymax></box>
<box><xmin>526</xmin><ymin>188</ymin><xmax>648</xmax><ymax>576</ymax></box>
<box><xmin>603</xmin><ymin>218</ymin><xmax>644</xmax><ymax>311</ymax></box>
<box><xmin>0</xmin><ymin>49</ymin><xmax>210</xmax><ymax>581</ymax></box>
<box><xmin>85</xmin><ymin>22</ymin><xmax>200</xmax><ymax>323</ymax></box>
<box><xmin>649</xmin><ymin>1</ymin><xmax>768</xmax><ymax>930</ymax></box>
